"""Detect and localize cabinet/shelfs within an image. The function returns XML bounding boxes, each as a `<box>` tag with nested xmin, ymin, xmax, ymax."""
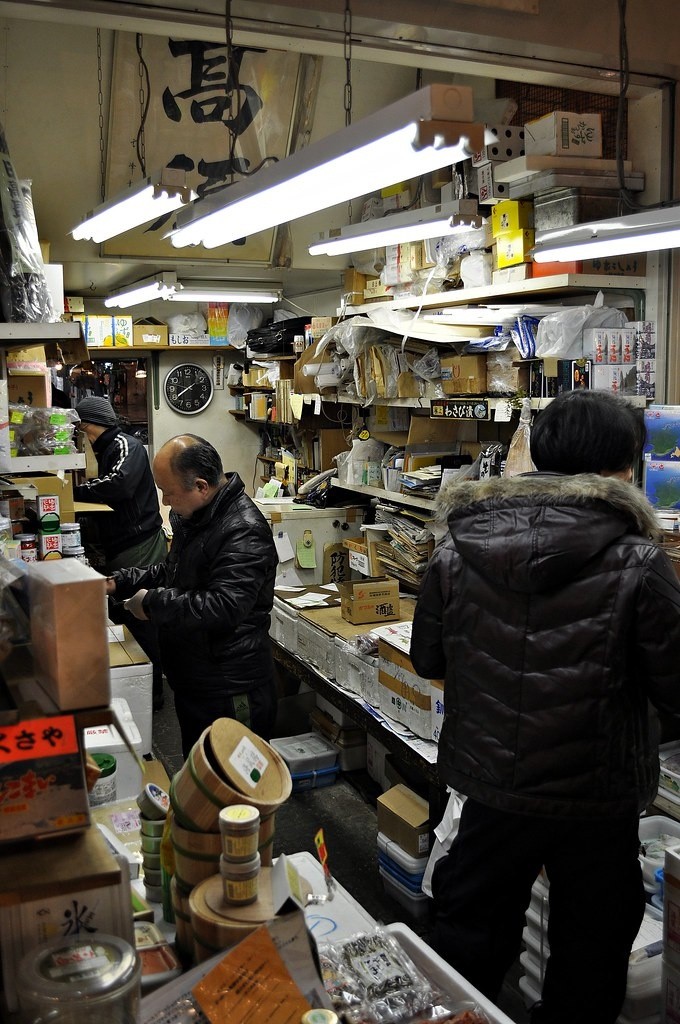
<box><xmin>0</xmin><ymin>320</ymin><xmax>113</xmax><ymax>731</ymax></box>
<box><xmin>329</xmin><ymin>249</ymin><xmax>668</xmax><ymax>593</ymax></box>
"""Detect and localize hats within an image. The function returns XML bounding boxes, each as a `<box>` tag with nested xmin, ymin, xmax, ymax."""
<box><xmin>75</xmin><ymin>396</ymin><xmax>117</xmax><ymax>426</ymax></box>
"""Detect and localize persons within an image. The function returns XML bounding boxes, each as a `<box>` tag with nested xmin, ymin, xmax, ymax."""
<box><xmin>103</xmin><ymin>433</ymin><xmax>278</xmax><ymax>764</ymax></box>
<box><xmin>411</xmin><ymin>391</ymin><xmax>680</xmax><ymax>1024</ymax></box>
<box><xmin>75</xmin><ymin>397</ymin><xmax>168</xmax><ymax>572</ymax></box>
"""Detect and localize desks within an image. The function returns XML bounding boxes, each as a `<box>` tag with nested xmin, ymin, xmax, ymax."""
<box><xmin>269</xmin><ymin>635</ymin><xmax>444</xmax><ymax>870</ymax></box>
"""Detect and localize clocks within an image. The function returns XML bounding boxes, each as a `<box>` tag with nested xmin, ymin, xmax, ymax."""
<box><xmin>163</xmin><ymin>363</ymin><xmax>214</xmax><ymax>415</ymax></box>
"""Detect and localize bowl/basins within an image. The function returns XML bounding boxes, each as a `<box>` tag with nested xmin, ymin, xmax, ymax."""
<box><xmin>135</xmin><ymin>783</ymin><xmax>169</xmax><ymax>901</ymax></box>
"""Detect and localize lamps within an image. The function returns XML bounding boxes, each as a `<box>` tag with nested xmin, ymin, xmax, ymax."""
<box><xmin>104</xmin><ymin>270</ymin><xmax>183</xmax><ymax>309</ymax></box>
<box><xmin>162</xmin><ymin>281</ymin><xmax>285</xmax><ymax>305</ymax></box>
<box><xmin>309</xmin><ymin>69</ymin><xmax>481</xmax><ymax>258</ymax></box>
<box><xmin>524</xmin><ymin>1</ymin><xmax>679</xmax><ymax>264</ymax></box>
<box><xmin>135</xmin><ymin>358</ymin><xmax>146</xmax><ymax>379</ymax></box>
<box><xmin>66</xmin><ymin>169</ymin><xmax>199</xmax><ymax>243</ymax></box>
<box><xmin>159</xmin><ymin>1</ymin><xmax>501</xmax><ymax>251</ymax></box>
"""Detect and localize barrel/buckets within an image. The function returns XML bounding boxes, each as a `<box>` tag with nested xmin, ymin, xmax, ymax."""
<box><xmin>166</xmin><ymin>717</ymin><xmax>294</xmax><ymax>973</ymax></box>
<box><xmin>12</xmin><ymin>932</ymin><xmax>144</xmax><ymax>1024</ymax></box>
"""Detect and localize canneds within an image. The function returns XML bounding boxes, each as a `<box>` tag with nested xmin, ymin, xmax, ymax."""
<box><xmin>218</xmin><ymin>804</ymin><xmax>261</xmax><ymax>907</ymax></box>
<box><xmin>15</xmin><ymin>522</ymin><xmax>85</xmax><ymax>564</ymax></box>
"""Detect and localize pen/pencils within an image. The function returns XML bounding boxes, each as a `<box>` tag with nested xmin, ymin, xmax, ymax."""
<box><xmin>106</xmin><ymin>573</ymin><xmax>122</xmax><ymax>582</ymax></box>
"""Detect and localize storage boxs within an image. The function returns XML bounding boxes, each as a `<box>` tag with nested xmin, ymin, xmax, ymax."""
<box><xmin>0</xmin><ymin>110</ymin><xmax>680</xmax><ymax>1024</ymax></box>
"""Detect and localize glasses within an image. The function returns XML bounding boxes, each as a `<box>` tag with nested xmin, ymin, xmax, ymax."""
<box><xmin>78</xmin><ymin>422</ymin><xmax>91</xmax><ymax>432</ymax></box>
